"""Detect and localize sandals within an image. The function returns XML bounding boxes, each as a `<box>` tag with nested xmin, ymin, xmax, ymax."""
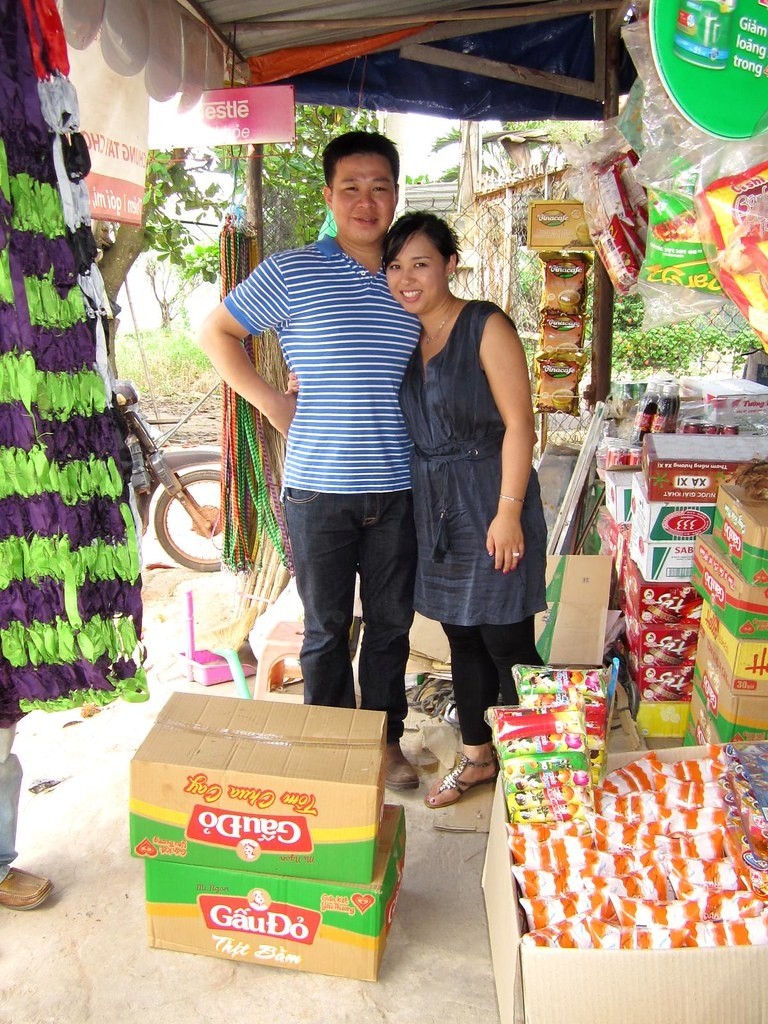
<box><xmin>423</xmin><ymin>745</ymin><xmax>500</xmax><ymax>808</ymax></box>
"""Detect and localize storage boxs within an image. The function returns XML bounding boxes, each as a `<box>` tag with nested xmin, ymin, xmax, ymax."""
<box><xmin>406</xmin><ymin>377</ymin><xmax>768</xmax><ymax>1023</ymax></box>
<box><xmin>144</xmin><ymin>805</ymin><xmax>406</xmax><ymax>982</ymax></box>
<box><xmin>129</xmin><ymin>689</ymin><xmax>389</xmax><ymax>886</ymax></box>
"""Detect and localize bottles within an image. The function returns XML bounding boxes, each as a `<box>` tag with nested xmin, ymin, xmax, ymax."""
<box><xmin>629</xmin><ymin>379</ymin><xmax>680</xmax><ymax>446</ymax></box>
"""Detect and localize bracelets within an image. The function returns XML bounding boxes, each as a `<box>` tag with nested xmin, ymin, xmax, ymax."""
<box><xmin>499</xmin><ymin>494</ymin><xmax>526</xmax><ymax>503</ymax></box>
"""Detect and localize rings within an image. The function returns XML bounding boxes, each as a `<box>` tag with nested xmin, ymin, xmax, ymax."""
<box><xmin>512</xmin><ymin>553</ymin><xmax>519</xmax><ymax>556</ymax></box>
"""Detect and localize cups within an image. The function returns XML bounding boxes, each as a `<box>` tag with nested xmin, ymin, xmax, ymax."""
<box><xmin>557</xmin><ymin>343</ymin><xmax>578</xmax><ymax>354</ymax></box>
<box><xmin>575</xmin><ymin>224</ymin><xmax>594</xmax><ymax>244</ymax></box>
<box><xmin>557</xmin><ymin>290</ymin><xmax>581</xmax><ymax>315</ymax></box>
<box><xmin>552</xmin><ymin>389</ymin><xmax>574</xmax><ymax>412</ymax></box>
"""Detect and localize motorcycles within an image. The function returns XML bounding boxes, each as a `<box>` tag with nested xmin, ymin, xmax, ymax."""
<box><xmin>113</xmin><ymin>380</ymin><xmax>229</xmax><ymax>573</ymax></box>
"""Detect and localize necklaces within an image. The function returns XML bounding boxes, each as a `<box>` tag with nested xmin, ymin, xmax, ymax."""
<box><xmin>416</xmin><ymin>297</ymin><xmax>459</xmax><ymax>345</ymax></box>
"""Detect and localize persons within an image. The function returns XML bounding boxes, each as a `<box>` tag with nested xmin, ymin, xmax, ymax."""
<box><xmin>199</xmin><ymin>132</ymin><xmax>422</xmax><ymax>789</ymax></box>
<box><xmin>286</xmin><ymin>213</ymin><xmax>548</xmax><ymax>807</ymax></box>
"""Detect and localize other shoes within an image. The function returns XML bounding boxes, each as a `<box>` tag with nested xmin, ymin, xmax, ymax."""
<box><xmin>383</xmin><ymin>742</ymin><xmax>421</xmax><ymax>791</ymax></box>
<box><xmin>1</xmin><ymin>867</ymin><xmax>54</xmax><ymax>912</ymax></box>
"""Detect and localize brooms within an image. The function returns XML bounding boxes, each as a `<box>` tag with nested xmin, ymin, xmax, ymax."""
<box><xmin>202</xmin><ymin>605</ymin><xmax>263</xmax><ymax>702</ymax></box>
